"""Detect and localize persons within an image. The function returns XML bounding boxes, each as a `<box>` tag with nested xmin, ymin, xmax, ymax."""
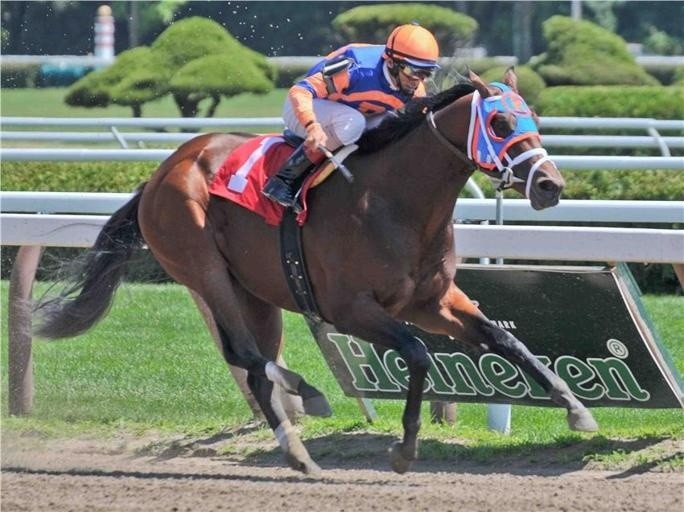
<box><xmin>257</xmin><ymin>20</ymin><xmax>443</xmax><ymax>209</ymax></box>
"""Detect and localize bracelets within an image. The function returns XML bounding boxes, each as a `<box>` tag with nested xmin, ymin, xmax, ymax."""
<box><xmin>303</xmin><ymin>119</ymin><xmax>322</xmax><ymax>128</ymax></box>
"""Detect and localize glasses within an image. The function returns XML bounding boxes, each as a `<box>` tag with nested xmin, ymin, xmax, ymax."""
<box><xmin>403</xmin><ymin>66</ymin><xmax>425</xmax><ymax>80</ymax></box>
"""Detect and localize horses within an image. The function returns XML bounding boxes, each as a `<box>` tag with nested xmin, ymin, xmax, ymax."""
<box><xmin>14</xmin><ymin>63</ymin><xmax>599</xmax><ymax>482</ymax></box>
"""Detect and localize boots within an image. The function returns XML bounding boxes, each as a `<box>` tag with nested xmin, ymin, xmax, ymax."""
<box><xmin>261</xmin><ymin>145</ymin><xmax>316</xmax><ymax>212</ymax></box>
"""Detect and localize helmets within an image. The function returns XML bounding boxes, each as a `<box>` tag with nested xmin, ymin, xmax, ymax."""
<box><xmin>383</xmin><ymin>23</ymin><xmax>440</xmax><ymax>69</ymax></box>
